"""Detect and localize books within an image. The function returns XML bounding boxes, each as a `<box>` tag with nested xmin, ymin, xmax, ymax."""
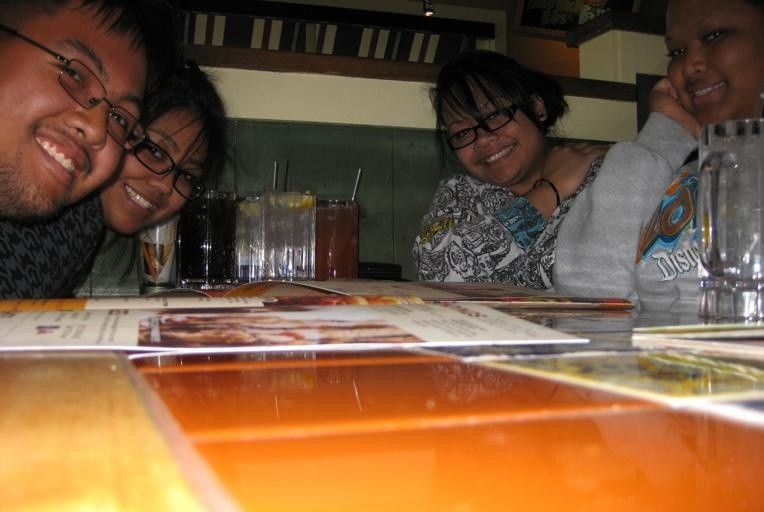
<box><xmin>249</xmin><ymin>17</ymin><xmax>283</xmax><ymax>50</ymax></box>
<box><xmin>631</xmin><ymin>326</ymin><xmax>763</xmax><ymax>361</ymax></box>
<box><xmin>193</xmin><ymin>14</ymin><xmax>226</xmax><ymax>47</ymax></box>
<box><xmin>408</xmin><ymin>33</ymin><xmax>441</xmax><ymax>64</ymax></box>
<box><xmin>1</xmin><ymin>291</ymin><xmax>591</xmax><ymax>363</ymax></box>
<box><xmin>476</xmin><ymin>38</ymin><xmax>497</xmax><ymax>51</ymax></box>
<box><xmin>484</xmin><ymin>298</ymin><xmax>632</xmax><ymax>306</ymax></box>
<box><xmin>291</xmin><ymin>22</ymin><xmax>337</xmax><ymax>55</ymax></box>
<box><xmin>358</xmin><ymin>29</ymin><xmax>390</xmax><ymax>59</ymax></box>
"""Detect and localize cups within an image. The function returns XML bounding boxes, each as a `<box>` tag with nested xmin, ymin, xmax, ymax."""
<box><xmin>134</xmin><ymin>189</ymin><xmax>360</xmax><ymax>292</ymax></box>
<box><xmin>697</xmin><ymin>117</ymin><xmax>764</xmax><ymax>324</ymax></box>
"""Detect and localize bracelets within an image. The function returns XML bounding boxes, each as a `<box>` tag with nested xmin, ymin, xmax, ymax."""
<box><xmin>533</xmin><ymin>179</ymin><xmax>560</xmax><ymax>207</ymax></box>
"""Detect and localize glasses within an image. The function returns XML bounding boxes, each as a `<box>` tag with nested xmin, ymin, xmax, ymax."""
<box><xmin>135</xmin><ymin>138</ymin><xmax>205</xmax><ymax>200</ymax></box>
<box><xmin>447</xmin><ymin>103</ymin><xmax>518</xmax><ymax>149</ymax></box>
<box><xmin>3</xmin><ymin>23</ymin><xmax>148</xmax><ymax>150</ymax></box>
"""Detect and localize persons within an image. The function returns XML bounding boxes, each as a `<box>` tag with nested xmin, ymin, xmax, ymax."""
<box><xmin>1</xmin><ymin>2</ymin><xmax>185</xmax><ymax>299</ymax></box>
<box><xmin>552</xmin><ymin>0</ymin><xmax>763</xmax><ymax>314</ymax></box>
<box><xmin>411</xmin><ymin>50</ymin><xmax>613</xmax><ymax>294</ymax></box>
<box><xmin>71</xmin><ymin>60</ymin><xmax>227</xmax><ymax>298</ymax></box>
